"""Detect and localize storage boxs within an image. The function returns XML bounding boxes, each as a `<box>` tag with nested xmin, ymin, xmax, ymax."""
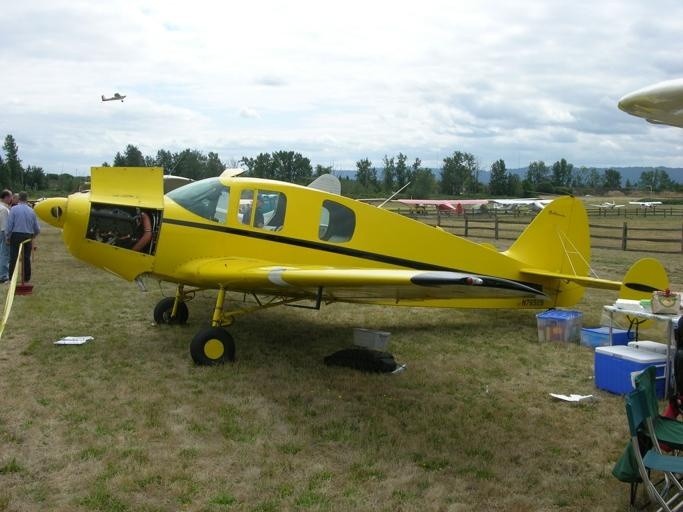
<box><xmin>535</xmin><ymin>309</ymin><xmax>584</xmax><ymax>344</ymax></box>
<box><xmin>628</xmin><ymin>340</ymin><xmax>676</xmax><ymax>393</ymax></box>
<box><xmin>594</xmin><ymin>345</ymin><xmax>672</xmax><ymax>400</ymax></box>
<box><xmin>352</xmin><ymin>328</ymin><xmax>391</xmax><ymax>353</ymax></box>
<box><xmin>580</xmin><ymin>326</ymin><xmax>634</xmax><ymax>350</ymax></box>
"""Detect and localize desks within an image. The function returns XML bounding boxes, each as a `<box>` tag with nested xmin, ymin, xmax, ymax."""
<box><xmin>603</xmin><ymin>305</ymin><xmax>680</xmax><ymax>401</ymax></box>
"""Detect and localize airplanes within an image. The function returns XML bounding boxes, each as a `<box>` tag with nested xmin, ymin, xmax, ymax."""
<box><xmin>102</xmin><ymin>93</ymin><xmax>126</xmax><ymax>102</ymax></box>
<box><xmin>34</xmin><ymin>166</ymin><xmax>669</xmax><ymax>364</ymax></box>
<box><xmin>398</xmin><ymin>198</ymin><xmax>663</xmax><ymax>217</ymax></box>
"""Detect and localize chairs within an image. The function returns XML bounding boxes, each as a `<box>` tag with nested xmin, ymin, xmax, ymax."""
<box><xmin>625</xmin><ymin>366</ymin><xmax>683</xmax><ymax>512</ymax></box>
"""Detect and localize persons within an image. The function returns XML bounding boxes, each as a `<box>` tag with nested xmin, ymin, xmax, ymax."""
<box><xmin>9</xmin><ymin>192</ymin><xmax>19</xmax><ymax>208</ymax></box>
<box><xmin>3</xmin><ymin>190</ymin><xmax>39</xmax><ymax>283</ymax></box>
<box><xmin>0</xmin><ymin>189</ymin><xmax>12</xmax><ymax>283</ymax></box>
<box><xmin>241</xmin><ymin>194</ymin><xmax>265</xmax><ymax>228</ymax></box>
